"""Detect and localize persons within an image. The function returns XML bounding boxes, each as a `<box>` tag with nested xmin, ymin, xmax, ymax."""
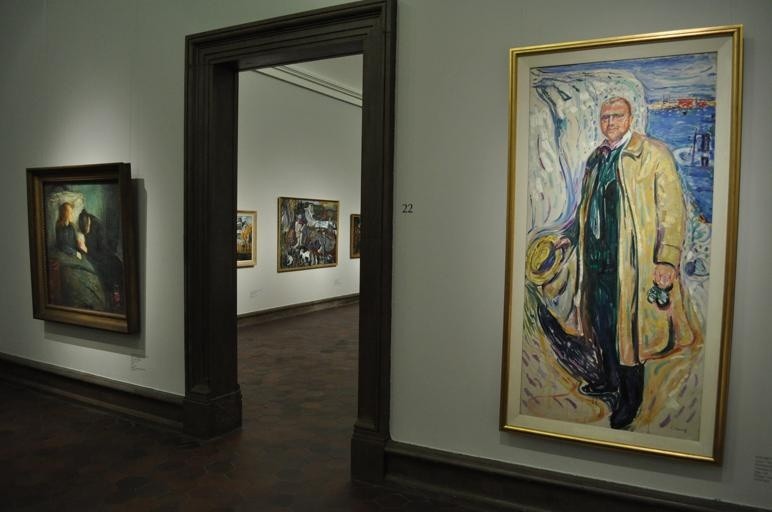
<box><xmin>76</xmin><ymin>207</ymin><xmax>125</xmax><ymax>294</ymax></box>
<box><xmin>54</xmin><ymin>202</ymin><xmax>83</xmax><ymax>260</ymax></box>
<box><xmin>542</xmin><ymin>98</ymin><xmax>701</xmax><ymax>432</ymax></box>
<box><xmin>291</xmin><ymin>213</ymin><xmax>306</xmax><ymax>248</ymax></box>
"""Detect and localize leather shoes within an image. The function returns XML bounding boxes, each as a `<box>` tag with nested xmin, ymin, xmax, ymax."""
<box><xmin>609</xmin><ymin>395</ymin><xmax>644</xmax><ymax>428</ymax></box>
<box><xmin>581</xmin><ymin>380</ymin><xmax>618</xmax><ymax>394</ymax></box>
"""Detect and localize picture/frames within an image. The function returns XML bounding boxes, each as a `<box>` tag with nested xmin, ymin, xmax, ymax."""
<box><xmin>349</xmin><ymin>213</ymin><xmax>365</xmax><ymax>260</ymax></box>
<box><xmin>236</xmin><ymin>210</ymin><xmax>258</xmax><ymax>269</ymax></box>
<box><xmin>26</xmin><ymin>162</ymin><xmax>138</xmax><ymax>335</ymax></box>
<box><xmin>498</xmin><ymin>23</ymin><xmax>744</xmax><ymax>467</ymax></box>
<box><xmin>276</xmin><ymin>197</ymin><xmax>340</xmax><ymax>273</ymax></box>
